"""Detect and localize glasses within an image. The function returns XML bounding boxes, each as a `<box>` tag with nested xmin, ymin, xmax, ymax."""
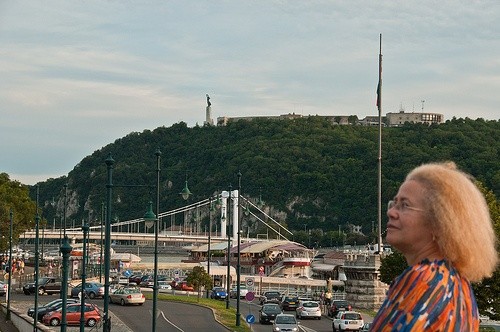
<box><xmin>387</xmin><ymin>200</ymin><xmax>428</xmax><ymax>214</ymax></box>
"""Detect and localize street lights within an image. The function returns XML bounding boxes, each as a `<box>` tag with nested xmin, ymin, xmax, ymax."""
<box><xmin>152</xmin><ymin>150</ymin><xmax>192</xmax><ymax>332</ymax></box>
<box><xmin>102</xmin><ymin>152</ymin><xmax>158</xmax><ymax>332</ymax></box>
<box><xmin>227</xmin><ymin>182</ymin><xmax>250</xmax><ymax>309</ymax></box>
<box><xmin>235</xmin><ymin>169</ymin><xmax>263</xmax><ymax>326</ymax></box>
<box><xmin>206</xmin><ymin>195</ymin><xmax>226</xmax><ymax>299</ymax></box>
<box><xmin>104</xmin><ymin>189</ymin><xmax>120</xmax><ymax>228</ymax></box>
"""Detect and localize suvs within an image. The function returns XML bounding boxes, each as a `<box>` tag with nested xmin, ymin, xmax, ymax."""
<box><xmin>23</xmin><ymin>277</ymin><xmax>61</xmax><ymax>296</ymax></box>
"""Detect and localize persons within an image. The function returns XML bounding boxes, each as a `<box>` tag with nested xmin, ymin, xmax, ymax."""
<box><xmin>367</xmin><ymin>162</ymin><xmax>500</xmax><ymax>332</ymax></box>
<box><xmin>321</xmin><ymin>289</ymin><xmax>332</xmax><ymax>307</ymax></box>
<box><xmin>46</xmin><ymin>262</ymin><xmax>63</xmax><ymax>274</ymax></box>
<box><xmin>0</xmin><ymin>255</ymin><xmax>25</xmax><ymax>278</ymax></box>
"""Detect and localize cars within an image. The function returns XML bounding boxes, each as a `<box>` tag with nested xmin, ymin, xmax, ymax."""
<box><xmin>326</xmin><ymin>299</ymin><xmax>373</xmax><ymax>332</ymax></box>
<box><xmin>270</xmin><ymin>314</ymin><xmax>300</xmax><ymax>332</ymax></box>
<box><xmin>258</xmin><ymin>291</ymin><xmax>321</xmax><ymax>325</ymax></box>
<box><xmin>0</xmin><ymin>282</ymin><xmax>6</xmax><ymax>295</ymax></box>
<box><xmin>28</xmin><ymin>298</ymin><xmax>101</xmax><ymax>327</ymax></box>
<box><xmin>211</xmin><ymin>287</ymin><xmax>227</xmax><ymax>300</ymax></box>
<box><xmin>230</xmin><ymin>284</ymin><xmax>249</xmax><ymax>299</ymax></box>
<box><xmin>71</xmin><ymin>282</ymin><xmax>145</xmax><ymax>306</ymax></box>
<box><xmin>110</xmin><ymin>271</ymin><xmax>193</xmax><ymax>291</ymax></box>
